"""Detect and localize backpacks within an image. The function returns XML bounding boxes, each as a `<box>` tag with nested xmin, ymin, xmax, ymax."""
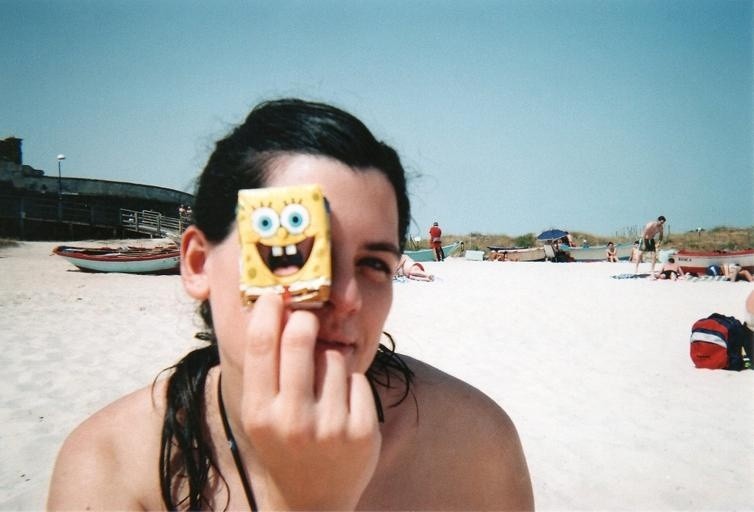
<box><xmin>690</xmin><ymin>313</ymin><xmax>754</xmax><ymax>371</ymax></box>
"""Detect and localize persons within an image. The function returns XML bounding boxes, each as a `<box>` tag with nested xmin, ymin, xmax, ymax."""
<box><xmin>606</xmin><ymin>241</ymin><xmax>620</xmax><ymax>263</ymax></box>
<box><xmin>186</xmin><ymin>205</ymin><xmax>192</xmax><ymax>219</ymax></box>
<box><xmin>744</xmin><ymin>290</ymin><xmax>754</xmax><ymax>333</ymax></box>
<box><xmin>634</xmin><ymin>215</ymin><xmax>668</xmax><ymax>274</ymax></box>
<box><xmin>429</xmin><ymin>220</ymin><xmax>445</xmax><ymax>262</ymax></box>
<box><xmin>718</xmin><ymin>261</ymin><xmax>754</xmax><ymax>285</ymax></box>
<box><xmin>178</xmin><ymin>202</ymin><xmax>187</xmax><ymax>227</ymax></box>
<box><xmin>629</xmin><ymin>239</ymin><xmax>645</xmax><ymax>263</ymax></box>
<box><xmin>46</xmin><ymin>96</ymin><xmax>537</xmax><ymax>512</ymax></box>
<box><xmin>395</xmin><ymin>252</ymin><xmax>437</xmax><ymax>284</ymax></box>
<box><xmin>655</xmin><ymin>257</ymin><xmax>685</xmax><ymax>282</ymax></box>
<box><xmin>546</xmin><ymin>236</ymin><xmax>575</xmax><ymax>264</ymax></box>
<box><xmin>487</xmin><ymin>248</ymin><xmax>505</xmax><ymax>262</ymax></box>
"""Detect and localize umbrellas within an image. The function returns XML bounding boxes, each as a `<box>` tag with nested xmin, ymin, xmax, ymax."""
<box><xmin>537</xmin><ymin>228</ymin><xmax>568</xmax><ymax>242</ymax></box>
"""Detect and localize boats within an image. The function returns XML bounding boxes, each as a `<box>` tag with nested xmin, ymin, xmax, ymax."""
<box><xmin>669</xmin><ymin>249</ymin><xmax>753</xmax><ymax>273</ymax></box>
<box><xmin>52</xmin><ymin>245</ymin><xmax>180</xmax><ymax>273</ymax></box>
<box><xmin>484</xmin><ymin>245</ymin><xmax>545</xmax><ymax>261</ymax></box>
<box><xmin>559</xmin><ymin>243</ymin><xmax>634</xmax><ymax>261</ymax></box>
<box><xmin>402</xmin><ymin>241</ymin><xmax>464</xmax><ymax>262</ymax></box>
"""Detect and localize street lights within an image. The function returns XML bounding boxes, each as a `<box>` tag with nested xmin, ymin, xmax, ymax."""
<box><xmin>57</xmin><ymin>154</ymin><xmax>65</xmax><ymax>201</ymax></box>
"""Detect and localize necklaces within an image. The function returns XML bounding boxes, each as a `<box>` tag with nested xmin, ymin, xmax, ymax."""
<box><xmin>214</xmin><ymin>372</ymin><xmax>258</xmax><ymax>512</ymax></box>
